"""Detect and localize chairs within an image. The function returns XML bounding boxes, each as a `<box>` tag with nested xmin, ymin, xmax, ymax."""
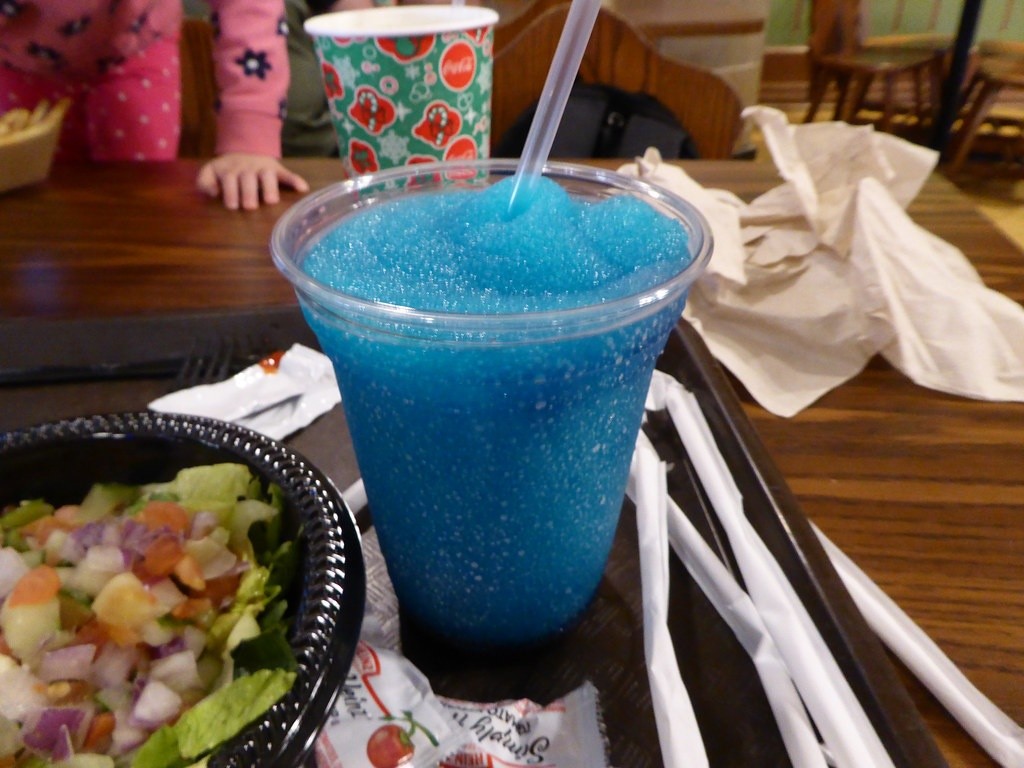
<box><xmin>803</xmin><ymin>0</ymin><xmax>1024</xmax><ymax>177</ymax></box>
<box><xmin>492</xmin><ymin>0</ymin><xmax>744</xmax><ymax>160</ymax></box>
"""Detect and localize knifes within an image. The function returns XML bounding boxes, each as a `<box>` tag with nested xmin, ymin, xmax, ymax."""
<box><xmin>0</xmin><ymin>354</ymin><xmax>260</xmax><ymax>388</ymax></box>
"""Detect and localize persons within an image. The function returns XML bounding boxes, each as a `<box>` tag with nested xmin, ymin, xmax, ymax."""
<box><xmin>0</xmin><ymin>1</ymin><xmax>410</xmax><ymax>212</ymax></box>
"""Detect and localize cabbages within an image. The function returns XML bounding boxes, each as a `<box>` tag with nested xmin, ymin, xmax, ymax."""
<box><xmin>113</xmin><ymin>462</ymin><xmax>300</xmax><ymax>768</ymax></box>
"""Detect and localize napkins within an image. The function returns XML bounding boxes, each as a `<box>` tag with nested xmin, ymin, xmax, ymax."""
<box><xmin>618</xmin><ymin>105</ymin><xmax>1024</xmax><ymax>419</ymax></box>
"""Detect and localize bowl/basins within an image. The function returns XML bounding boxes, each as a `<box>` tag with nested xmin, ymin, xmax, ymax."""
<box><xmin>0</xmin><ymin>408</ymin><xmax>367</xmax><ymax>768</ymax></box>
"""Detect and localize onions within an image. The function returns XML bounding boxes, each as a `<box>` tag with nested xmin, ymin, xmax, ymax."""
<box><xmin>0</xmin><ymin>515</ymin><xmax>255</xmax><ymax>763</ymax></box>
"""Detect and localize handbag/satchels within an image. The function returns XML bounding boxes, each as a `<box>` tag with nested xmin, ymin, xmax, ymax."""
<box><xmin>492</xmin><ymin>81</ymin><xmax>700</xmax><ymax>162</ymax></box>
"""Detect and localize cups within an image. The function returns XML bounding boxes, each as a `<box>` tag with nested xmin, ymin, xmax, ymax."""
<box><xmin>270</xmin><ymin>157</ymin><xmax>715</xmax><ymax>655</ymax></box>
<box><xmin>302</xmin><ymin>4</ymin><xmax>499</xmax><ymax>204</ymax></box>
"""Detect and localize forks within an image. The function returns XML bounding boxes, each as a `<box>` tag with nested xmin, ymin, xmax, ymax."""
<box><xmin>170</xmin><ymin>327</ymin><xmax>235</xmax><ymax>393</ymax></box>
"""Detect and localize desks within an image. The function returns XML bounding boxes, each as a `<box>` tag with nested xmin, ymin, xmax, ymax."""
<box><xmin>0</xmin><ymin>157</ymin><xmax>1024</xmax><ymax>767</ymax></box>
<box><xmin>621</xmin><ymin>1</ymin><xmax>766</xmax><ymax>36</ymax></box>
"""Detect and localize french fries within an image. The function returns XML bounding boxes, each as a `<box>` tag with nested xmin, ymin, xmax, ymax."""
<box><xmin>0</xmin><ymin>99</ymin><xmax>48</xmax><ymax>141</ymax></box>
